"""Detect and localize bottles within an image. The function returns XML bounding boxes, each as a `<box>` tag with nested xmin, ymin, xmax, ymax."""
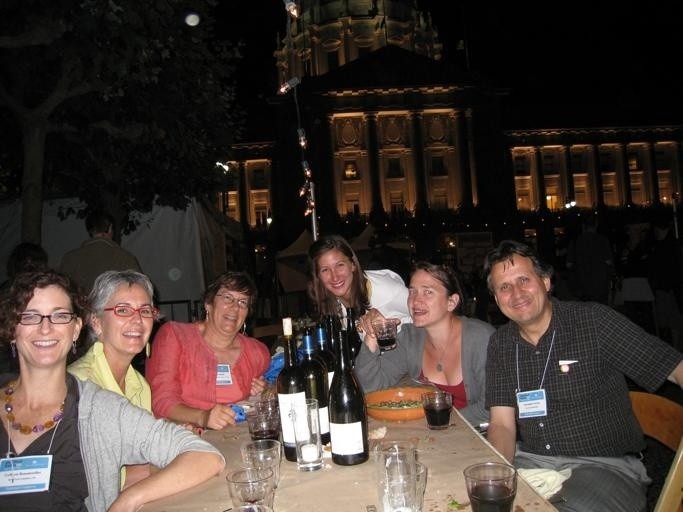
<box><xmin>242</xmin><ymin>301</ymin><xmax>370</xmax><ymax>472</ymax></box>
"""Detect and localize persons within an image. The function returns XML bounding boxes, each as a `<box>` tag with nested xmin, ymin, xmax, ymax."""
<box><xmin>349</xmin><ymin>262</ymin><xmax>498</xmax><ymax>439</ymax></box>
<box><xmin>479</xmin><ymin>238</ymin><xmax>683</xmax><ymax>512</ymax></box>
<box><xmin>307</xmin><ymin>234</ymin><xmax>414</xmax><ymax>333</ymax></box>
<box><xmin>57</xmin><ymin>209</ymin><xmax>145</xmax><ymax>354</ymax></box>
<box><xmin>144</xmin><ymin>267</ymin><xmax>272</xmax><ymax>438</ymax></box>
<box><xmin>65</xmin><ymin>268</ymin><xmax>159</xmax><ymax>494</ymax></box>
<box><xmin>0</xmin><ymin>258</ymin><xmax>227</xmax><ymax>510</ymax></box>
<box><xmin>0</xmin><ymin>239</ymin><xmax>51</xmax><ymax>293</ymax></box>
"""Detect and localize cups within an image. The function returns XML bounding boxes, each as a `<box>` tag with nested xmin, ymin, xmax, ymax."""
<box><xmin>241</xmin><ymin>438</ymin><xmax>280</xmax><ymax>489</ymax></box>
<box><xmin>225</xmin><ymin>467</ymin><xmax>275</xmax><ymax>508</ymax></box>
<box><xmin>224</xmin><ymin>504</ymin><xmax>278</xmax><ymax>511</ymax></box>
<box><xmin>422</xmin><ymin>390</ymin><xmax>453</xmax><ymax>430</ymax></box>
<box><xmin>463</xmin><ymin>460</ymin><xmax>517</xmax><ymax>511</ymax></box>
<box><xmin>371</xmin><ymin>438</ymin><xmax>430</xmax><ymax>512</ymax></box>
<box><xmin>372</xmin><ymin>317</ymin><xmax>398</xmax><ymax>352</ymax></box>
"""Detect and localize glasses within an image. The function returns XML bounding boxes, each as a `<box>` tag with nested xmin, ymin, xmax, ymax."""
<box><xmin>214</xmin><ymin>292</ymin><xmax>252</xmax><ymax>309</ymax></box>
<box><xmin>104</xmin><ymin>305</ymin><xmax>159</xmax><ymax>319</ymax></box>
<box><xmin>15</xmin><ymin>312</ymin><xmax>78</xmax><ymax>326</ymax></box>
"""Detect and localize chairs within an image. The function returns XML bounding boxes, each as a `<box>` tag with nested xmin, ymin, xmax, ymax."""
<box><xmin>620</xmin><ymin>390</ymin><xmax>683</xmax><ymax>512</ymax></box>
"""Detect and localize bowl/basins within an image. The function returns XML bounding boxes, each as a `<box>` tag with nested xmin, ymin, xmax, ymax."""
<box><xmin>362</xmin><ymin>386</ymin><xmax>438</xmax><ymax>421</ymax></box>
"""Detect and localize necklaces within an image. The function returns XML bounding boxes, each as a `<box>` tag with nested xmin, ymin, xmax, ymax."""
<box><xmin>3</xmin><ymin>379</ymin><xmax>67</xmax><ymax>433</ymax></box>
<box><xmin>423</xmin><ymin>315</ymin><xmax>456</xmax><ymax>373</ymax></box>
